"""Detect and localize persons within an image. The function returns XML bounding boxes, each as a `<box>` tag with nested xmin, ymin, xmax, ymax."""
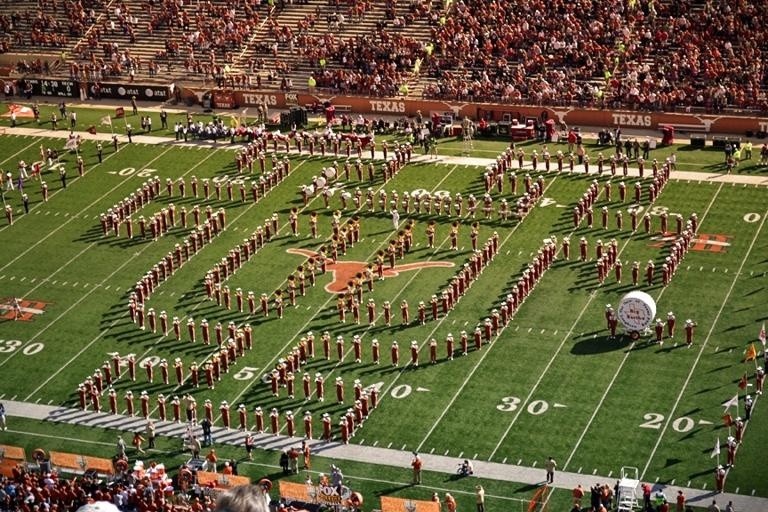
<box><xmin>3</xmin><ymin>1</ymin><xmax>768</xmax><ymax>512</ymax></box>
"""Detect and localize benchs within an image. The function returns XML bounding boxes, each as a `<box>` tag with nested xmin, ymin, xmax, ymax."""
<box><xmin>0</xmin><ymin>0</ymin><xmax>768</xmax><ymax>117</ymax></box>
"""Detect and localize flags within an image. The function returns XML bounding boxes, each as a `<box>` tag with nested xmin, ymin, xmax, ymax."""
<box><xmin>720</xmin><ymin>414</ymin><xmax>732</xmax><ymax>426</ymax></box>
<box><xmin>758</xmin><ymin>320</ymin><xmax>766</xmax><ymax>353</ymax></box>
<box><xmin>722</xmin><ymin>394</ymin><xmax>738</xmax><ymax>413</ymax></box>
<box><xmin>745</xmin><ymin>344</ymin><xmax>756</xmax><ymax>361</ymax></box>
<box><xmin>710</xmin><ymin>439</ymin><xmax>720</xmax><ymax>458</ymax></box>
<box><xmin>738</xmin><ymin>373</ymin><xmax>747</xmax><ymax>389</ymax></box>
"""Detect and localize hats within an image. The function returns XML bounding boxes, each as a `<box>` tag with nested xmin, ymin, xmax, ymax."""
<box><xmin>75</xmin><ymin>502</ymin><xmax>120</xmax><ymax>512</ymax></box>
